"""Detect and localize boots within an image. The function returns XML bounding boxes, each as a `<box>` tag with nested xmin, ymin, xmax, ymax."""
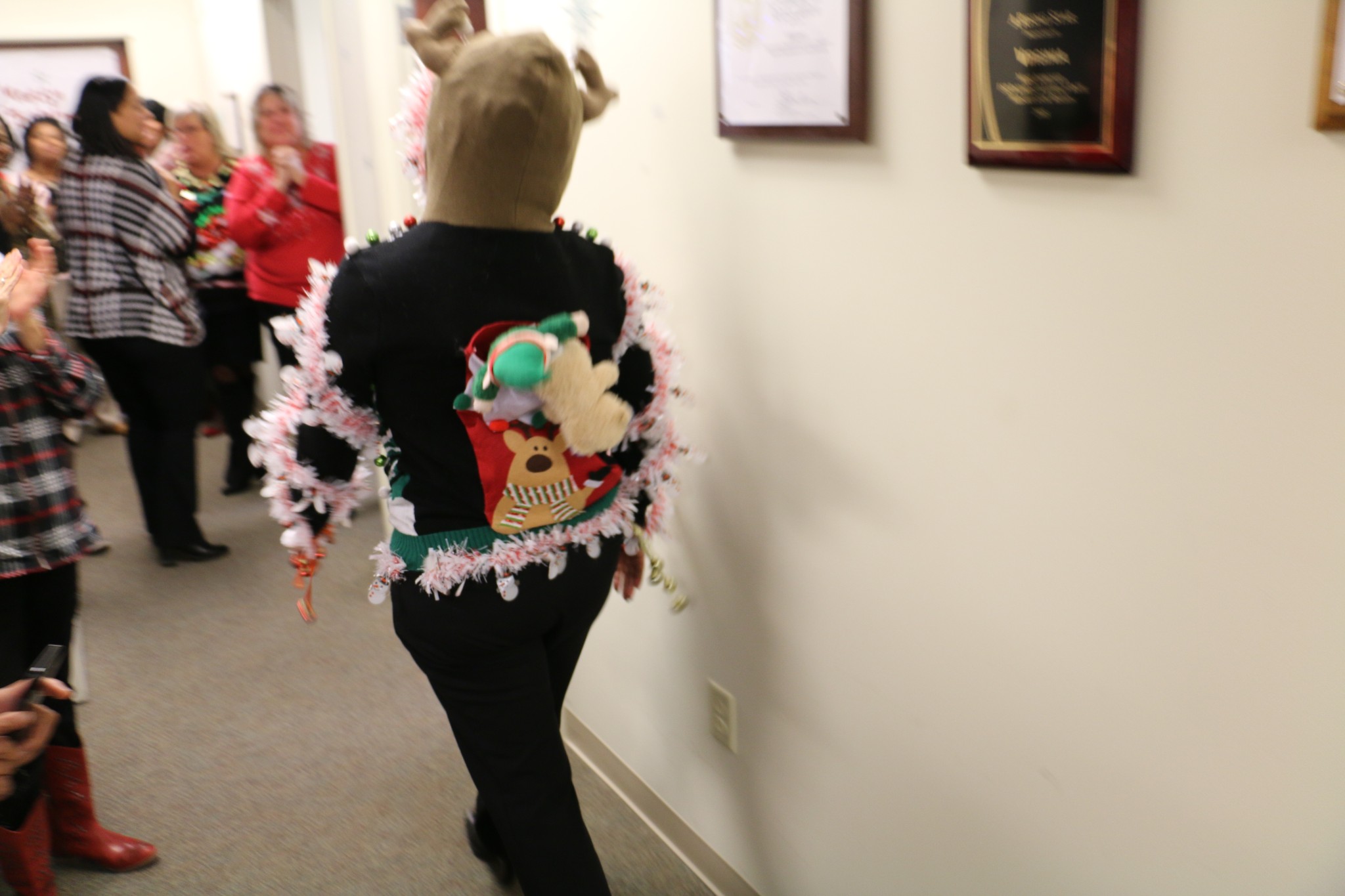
<box><xmin>0</xmin><ymin>797</ymin><xmax>60</xmax><ymax>896</ymax></box>
<box><xmin>45</xmin><ymin>733</ymin><xmax>158</xmax><ymax>872</ymax></box>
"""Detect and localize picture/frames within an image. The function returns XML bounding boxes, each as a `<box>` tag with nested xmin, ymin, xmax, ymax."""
<box><xmin>955</xmin><ymin>0</ymin><xmax>1143</xmax><ymax>175</ymax></box>
<box><xmin>706</xmin><ymin>0</ymin><xmax>873</xmax><ymax>146</ymax></box>
<box><xmin>1300</xmin><ymin>0</ymin><xmax>1345</xmax><ymax>131</ymax></box>
<box><xmin>0</xmin><ymin>34</ymin><xmax>140</xmax><ymax>178</ymax></box>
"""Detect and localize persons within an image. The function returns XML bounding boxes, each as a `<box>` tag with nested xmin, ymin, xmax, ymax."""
<box><xmin>0</xmin><ymin>96</ymin><xmax>175</xmax><ymax>268</ymax></box>
<box><xmin>221</xmin><ymin>83</ymin><xmax>344</xmax><ymax>339</ymax></box>
<box><xmin>283</xmin><ymin>1</ymin><xmax>675</xmax><ymax>895</ymax></box>
<box><xmin>60</xmin><ymin>75</ymin><xmax>233</xmax><ymax>565</ymax></box>
<box><xmin>164</xmin><ymin>106</ymin><xmax>267</xmax><ymax>494</ymax></box>
<box><xmin>0</xmin><ymin>240</ymin><xmax>162</xmax><ymax>896</ymax></box>
<box><xmin>21</xmin><ymin>114</ymin><xmax>127</xmax><ymax>446</ymax></box>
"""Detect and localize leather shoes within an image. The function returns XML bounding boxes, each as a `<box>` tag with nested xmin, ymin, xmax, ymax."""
<box><xmin>159</xmin><ymin>535</ymin><xmax>228</xmax><ymax>568</ymax></box>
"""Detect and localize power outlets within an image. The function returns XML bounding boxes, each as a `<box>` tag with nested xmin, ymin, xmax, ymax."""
<box><xmin>704</xmin><ymin>677</ymin><xmax>741</xmax><ymax>754</ymax></box>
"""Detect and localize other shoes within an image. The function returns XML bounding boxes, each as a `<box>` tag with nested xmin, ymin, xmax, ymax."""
<box><xmin>220</xmin><ymin>469</ymin><xmax>269</xmax><ymax>496</ymax></box>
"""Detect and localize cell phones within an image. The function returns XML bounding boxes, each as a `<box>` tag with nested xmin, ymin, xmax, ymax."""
<box><xmin>13</xmin><ymin>641</ymin><xmax>63</xmax><ymax>737</ymax></box>
